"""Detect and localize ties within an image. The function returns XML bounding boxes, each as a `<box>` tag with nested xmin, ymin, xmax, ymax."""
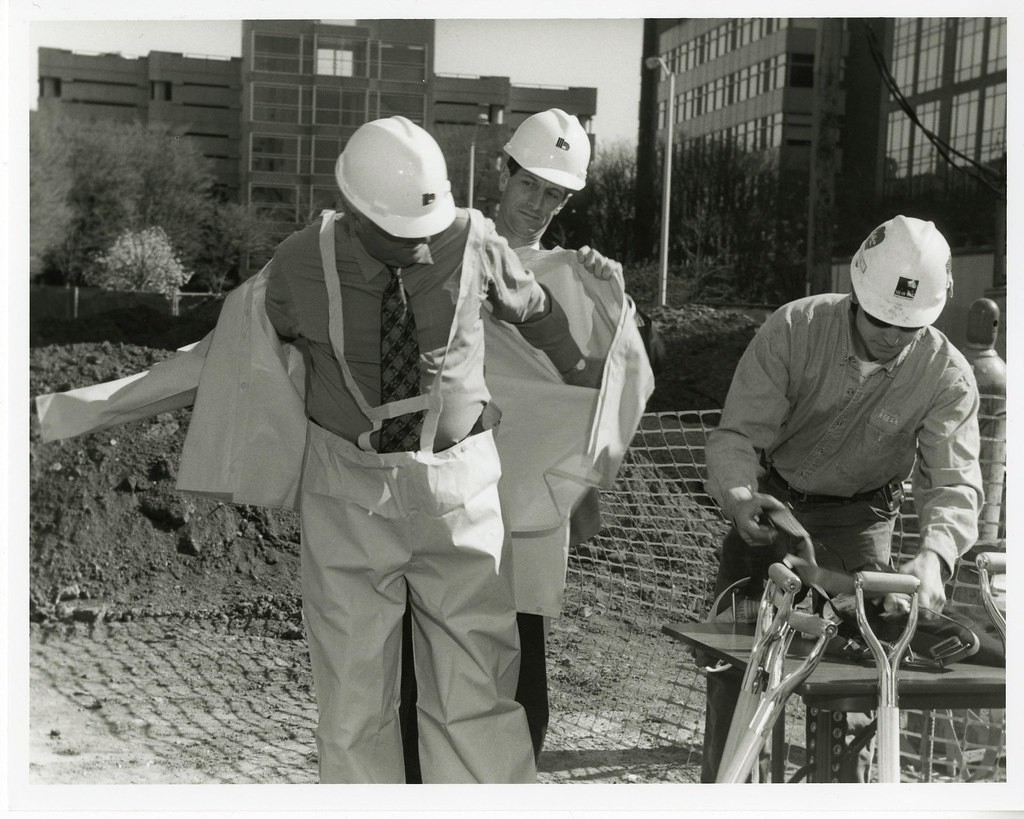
<box><xmin>378</xmin><ymin>265</ymin><xmax>423</xmax><ymax>454</ymax></box>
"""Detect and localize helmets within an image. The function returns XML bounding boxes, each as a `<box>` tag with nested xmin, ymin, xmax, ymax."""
<box><xmin>849</xmin><ymin>217</ymin><xmax>952</xmax><ymax>327</ymax></box>
<box><xmin>334</xmin><ymin>115</ymin><xmax>457</xmax><ymax>238</ymax></box>
<box><xmin>503</xmin><ymin>108</ymin><xmax>591</xmax><ymax>191</ymax></box>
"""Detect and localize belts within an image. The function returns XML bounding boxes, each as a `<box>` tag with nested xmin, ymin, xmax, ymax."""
<box><xmin>770</xmin><ymin>467</ymin><xmax>879</xmax><ymax>501</ymax></box>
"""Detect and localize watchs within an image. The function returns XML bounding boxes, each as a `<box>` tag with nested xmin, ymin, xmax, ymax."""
<box><xmin>576</xmin><ymin>358</ymin><xmax>587</xmax><ymax>371</ymax></box>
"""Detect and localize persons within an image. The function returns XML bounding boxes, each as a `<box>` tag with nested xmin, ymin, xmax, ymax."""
<box><xmin>37</xmin><ymin>108</ymin><xmax>653</xmax><ymax>784</ymax></box>
<box><xmin>699</xmin><ymin>215</ymin><xmax>983</xmax><ymax>784</ymax></box>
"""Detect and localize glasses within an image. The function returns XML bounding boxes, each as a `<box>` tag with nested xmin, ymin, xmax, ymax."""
<box><xmin>862</xmin><ymin>309</ymin><xmax>926</xmax><ymax>333</ymax></box>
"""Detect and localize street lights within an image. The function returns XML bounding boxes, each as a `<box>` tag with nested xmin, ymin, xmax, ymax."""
<box><xmin>645</xmin><ymin>56</ymin><xmax>676</xmax><ymax>306</ymax></box>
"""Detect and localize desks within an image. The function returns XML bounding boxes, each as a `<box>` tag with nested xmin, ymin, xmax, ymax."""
<box><xmin>660</xmin><ymin>624</ymin><xmax>1007</xmax><ymax>783</ymax></box>
